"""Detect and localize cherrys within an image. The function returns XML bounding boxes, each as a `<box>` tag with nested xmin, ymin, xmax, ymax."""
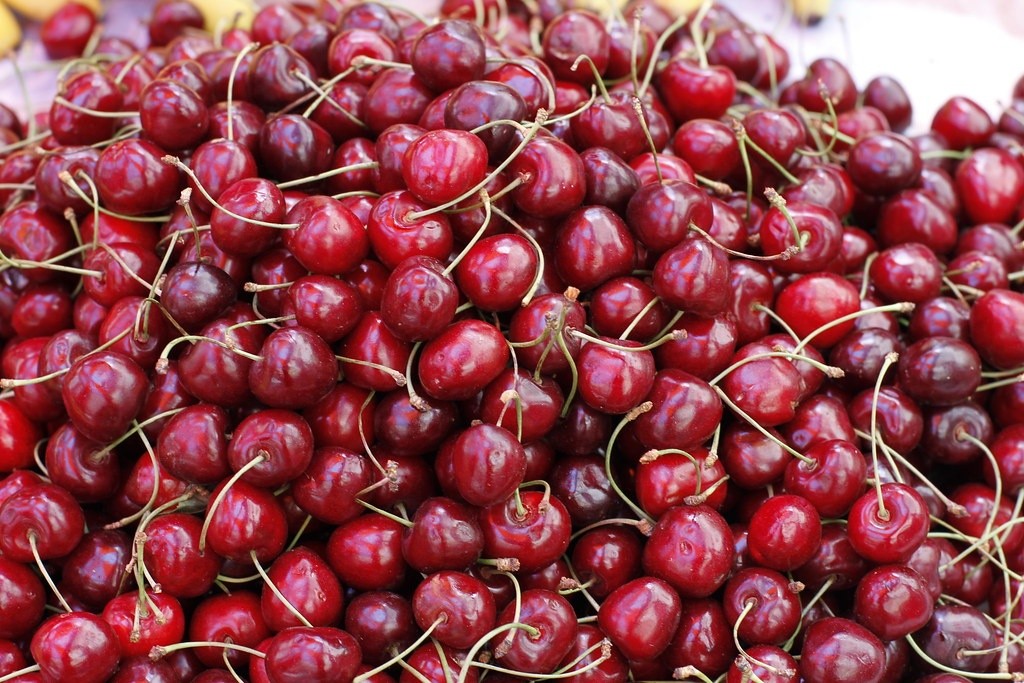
<box><xmin>0</xmin><ymin>0</ymin><xmax>1024</xmax><ymax>681</ymax></box>
